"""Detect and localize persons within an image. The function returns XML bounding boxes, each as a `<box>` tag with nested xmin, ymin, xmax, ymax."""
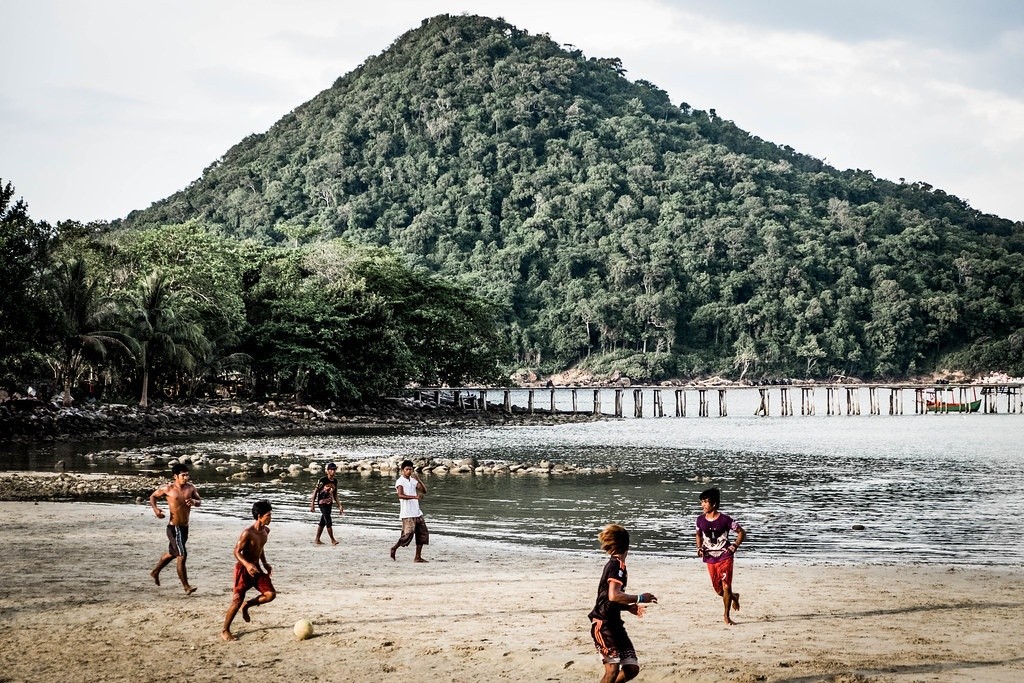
<box><xmin>588</xmin><ymin>526</ymin><xmax>658</xmax><ymax>683</ymax></box>
<box><xmin>222</xmin><ymin>500</ymin><xmax>276</xmax><ymax>640</ymax></box>
<box><xmin>150</xmin><ymin>464</ymin><xmax>202</xmax><ymax>595</ymax></box>
<box><xmin>391</xmin><ymin>460</ymin><xmax>431</xmax><ymax>563</ymax></box>
<box><xmin>695</xmin><ymin>488</ymin><xmax>746</xmax><ymax>625</ymax></box>
<box><xmin>311</xmin><ymin>463</ymin><xmax>344</xmax><ymax>546</ymax></box>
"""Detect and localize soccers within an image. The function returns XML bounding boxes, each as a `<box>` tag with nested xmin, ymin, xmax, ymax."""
<box><xmin>293</xmin><ymin>619</ymin><xmax>312</xmax><ymax>639</ymax></box>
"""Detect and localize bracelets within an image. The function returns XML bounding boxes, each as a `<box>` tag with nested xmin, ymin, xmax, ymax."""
<box><xmin>636</xmin><ymin>595</ymin><xmax>643</xmax><ymax>604</ymax></box>
<box><xmin>732</xmin><ymin>544</ymin><xmax>738</xmax><ymax>548</ymax></box>
